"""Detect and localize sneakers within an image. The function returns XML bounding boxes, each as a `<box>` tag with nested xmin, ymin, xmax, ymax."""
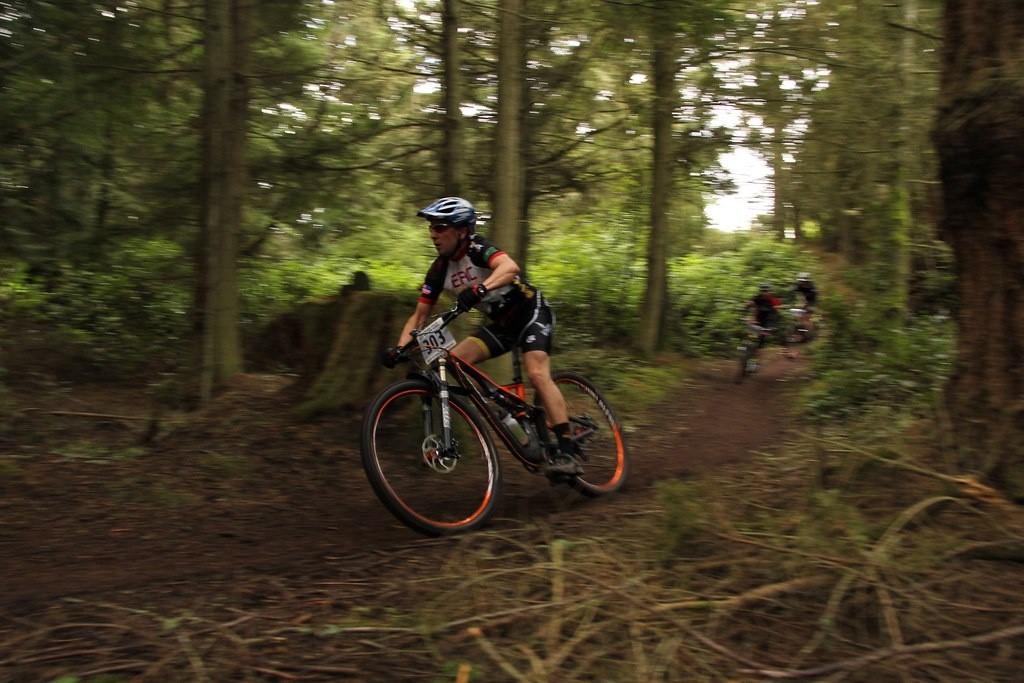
<box><xmin>545</xmin><ymin>455</ymin><xmax>584</xmax><ymax>478</ymax></box>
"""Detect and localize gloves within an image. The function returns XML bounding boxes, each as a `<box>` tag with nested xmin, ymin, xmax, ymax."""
<box><xmin>457</xmin><ymin>284</ymin><xmax>487</xmax><ymax>313</ymax></box>
<box><xmin>381</xmin><ymin>346</ymin><xmax>405</xmax><ymax>369</ymax></box>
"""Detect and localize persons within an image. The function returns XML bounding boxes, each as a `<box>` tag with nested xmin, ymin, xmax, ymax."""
<box><xmin>379</xmin><ymin>197</ymin><xmax>585</xmax><ymax>479</ymax></box>
<box><xmin>745</xmin><ymin>272</ymin><xmax>823</xmax><ymax>348</ymax></box>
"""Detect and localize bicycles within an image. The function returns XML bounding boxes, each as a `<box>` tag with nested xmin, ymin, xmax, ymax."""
<box><xmin>734</xmin><ymin>319</ymin><xmax>772</xmax><ymax>384</ymax></box>
<box><xmin>361</xmin><ymin>282</ymin><xmax>630</xmax><ymax>535</ymax></box>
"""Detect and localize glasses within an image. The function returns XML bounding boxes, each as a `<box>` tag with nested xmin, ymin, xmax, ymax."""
<box><xmin>429</xmin><ymin>223</ymin><xmax>453</xmax><ymax>233</ymax></box>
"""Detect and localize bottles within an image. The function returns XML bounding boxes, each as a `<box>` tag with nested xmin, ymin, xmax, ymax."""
<box><xmin>497</xmin><ymin>409</ymin><xmax>530</xmax><ymax>446</ymax></box>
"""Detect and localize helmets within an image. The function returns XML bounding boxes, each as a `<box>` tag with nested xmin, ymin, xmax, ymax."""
<box><xmin>416</xmin><ymin>197</ymin><xmax>477</xmax><ymax>235</ymax></box>
<box><xmin>759</xmin><ymin>284</ymin><xmax>771</xmax><ymax>291</ymax></box>
<box><xmin>796</xmin><ymin>272</ymin><xmax>811</xmax><ymax>281</ymax></box>
